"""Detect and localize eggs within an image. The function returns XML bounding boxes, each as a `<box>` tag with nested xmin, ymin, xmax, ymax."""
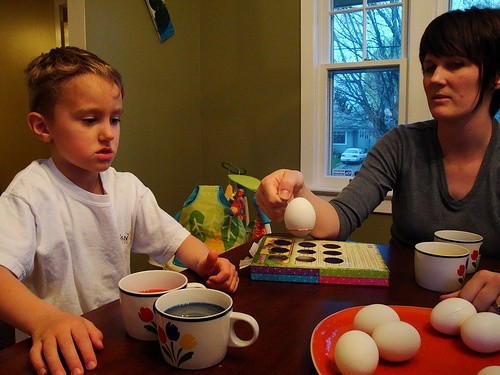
<box><xmin>334</xmin><ymin>329</ymin><xmax>379</xmax><ymax>375</ymax></box>
<box><xmin>372</xmin><ymin>321</ymin><xmax>421</xmax><ymax>361</ymax></box>
<box><xmin>477</xmin><ymin>365</ymin><xmax>500</xmax><ymax>375</ymax></box>
<box><xmin>284</xmin><ymin>197</ymin><xmax>316</xmax><ymax>237</ymax></box>
<box><xmin>460</xmin><ymin>312</ymin><xmax>500</xmax><ymax>353</ymax></box>
<box><xmin>430</xmin><ymin>297</ymin><xmax>477</xmax><ymax>335</ymax></box>
<box><xmin>354</xmin><ymin>304</ymin><xmax>400</xmax><ymax>336</ymax></box>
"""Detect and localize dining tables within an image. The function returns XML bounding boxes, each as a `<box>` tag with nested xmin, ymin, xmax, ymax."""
<box><xmin>1</xmin><ymin>233</ymin><xmax>500</xmax><ymax>375</ymax></box>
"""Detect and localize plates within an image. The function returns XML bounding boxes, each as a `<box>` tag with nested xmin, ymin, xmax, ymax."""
<box><xmin>309</xmin><ymin>303</ymin><xmax>500</xmax><ymax>375</ymax></box>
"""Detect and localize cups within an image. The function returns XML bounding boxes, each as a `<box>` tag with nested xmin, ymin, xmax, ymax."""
<box><xmin>414</xmin><ymin>242</ymin><xmax>468</xmax><ymax>292</ymax></box>
<box><xmin>119</xmin><ymin>270</ymin><xmax>206</xmax><ymax>341</ymax></box>
<box><xmin>434</xmin><ymin>229</ymin><xmax>482</xmax><ymax>275</ymax></box>
<box><xmin>154</xmin><ymin>287</ymin><xmax>259</xmax><ymax>369</ymax></box>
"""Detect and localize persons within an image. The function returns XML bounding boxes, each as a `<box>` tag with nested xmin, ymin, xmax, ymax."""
<box><xmin>0</xmin><ymin>46</ymin><xmax>240</xmax><ymax>375</ymax></box>
<box><xmin>255</xmin><ymin>7</ymin><xmax>500</xmax><ymax>316</ymax></box>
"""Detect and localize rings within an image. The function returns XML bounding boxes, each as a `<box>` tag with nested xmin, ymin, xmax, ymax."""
<box><xmin>492</xmin><ymin>301</ymin><xmax>500</xmax><ymax>313</ymax></box>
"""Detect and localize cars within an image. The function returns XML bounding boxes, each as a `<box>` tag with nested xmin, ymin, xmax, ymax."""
<box><xmin>341</xmin><ymin>148</ymin><xmax>367</xmax><ymax>164</ymax></box>
